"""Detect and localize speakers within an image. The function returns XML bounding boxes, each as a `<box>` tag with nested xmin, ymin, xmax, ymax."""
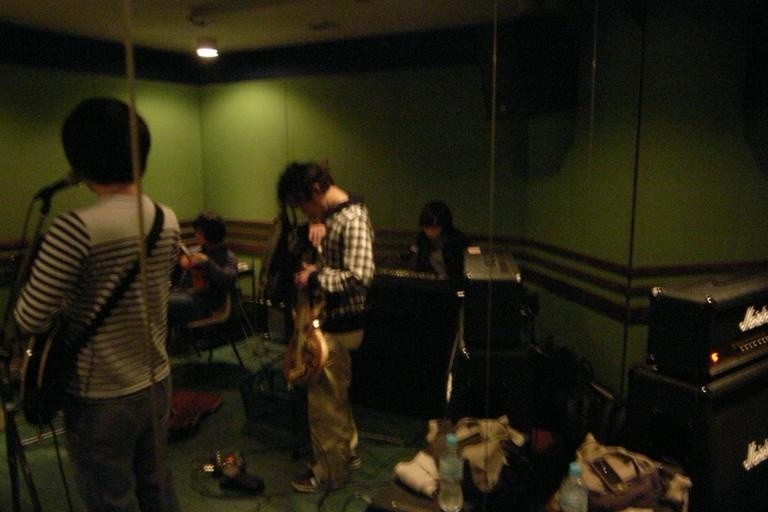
<box><xmin>621</xmin><ymin>356</ymin><xmax>768</xmax><ymax>512</ymax></box>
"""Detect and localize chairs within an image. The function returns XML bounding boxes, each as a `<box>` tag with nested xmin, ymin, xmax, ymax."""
<box><xmin>181</xmin><ymin>291</ymin><xmax>243</xmax><ymax>366</ymax></box>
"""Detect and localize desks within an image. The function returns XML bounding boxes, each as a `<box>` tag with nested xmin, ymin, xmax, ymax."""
<box><xmin>371</xmin><ymin>431</ymin><xmax>696</xmax><ymax>512</ymax></box>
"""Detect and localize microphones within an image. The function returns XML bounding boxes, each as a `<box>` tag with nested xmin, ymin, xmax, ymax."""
<box><xmin>36</xmin><ymin>170</ymin><xmax>87</xmax><ymax>197</ymax></box>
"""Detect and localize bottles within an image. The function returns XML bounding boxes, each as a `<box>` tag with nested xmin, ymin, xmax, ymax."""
<box><xmin>432</xmin><ymin>431</ymin><xmax>464</xmax><ymax>510</ymax></box>
<box><xmin>556</xmin><ymin>462</ymin><xmax>590</xmax><ymax>512</ymax></box>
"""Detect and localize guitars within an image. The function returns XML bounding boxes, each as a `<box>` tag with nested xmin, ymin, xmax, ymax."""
<box><xmin>21</xmin><ymin>318</ymin><xmax>65</xmax><ymax>425</ymax></box>
<box><xmin>283</xmin><ymin>157</ymin><xmax>330</xmax><ymax>390</ymax></box>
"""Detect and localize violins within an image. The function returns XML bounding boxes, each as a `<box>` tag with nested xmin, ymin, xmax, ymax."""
<box><xmin>178</xmin><ymin>237</ymin><xmax>208</xmax><ymax>291</ymax></box>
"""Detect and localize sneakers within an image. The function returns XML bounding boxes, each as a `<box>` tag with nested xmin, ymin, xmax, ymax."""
<box><xmin>215</xmin><ymin>468</ymin><xmax>267</xmax><ymax>498</ymax></box>
<box><xmin>289</xmin><ymin>469</ymin><xmax>348</xmax><ymax>493</ymax></box>
<box><xmin>346</xmin><ymin>451</ymin><xmax>363</xmax><ymax>470</ymax></box>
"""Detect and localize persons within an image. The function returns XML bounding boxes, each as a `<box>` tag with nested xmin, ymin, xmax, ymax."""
<box><xmin>12</xmin><ymin>95</ymin><xmax>182</xmax><ymax>510</ymax></box>
<box><xmin>168</xmin><ymin>210</ymin><xmax>239</xmax><ymax>320</ymax></box>
<box><xmin>398</xmin><ymin>200</ymin><xmax>469</xmax><ymax>273</ymax></box>
<box><xmin>277</xmin><ymin>157</ymin><xmax>378</xmax><ymax>495</ymax></box>
